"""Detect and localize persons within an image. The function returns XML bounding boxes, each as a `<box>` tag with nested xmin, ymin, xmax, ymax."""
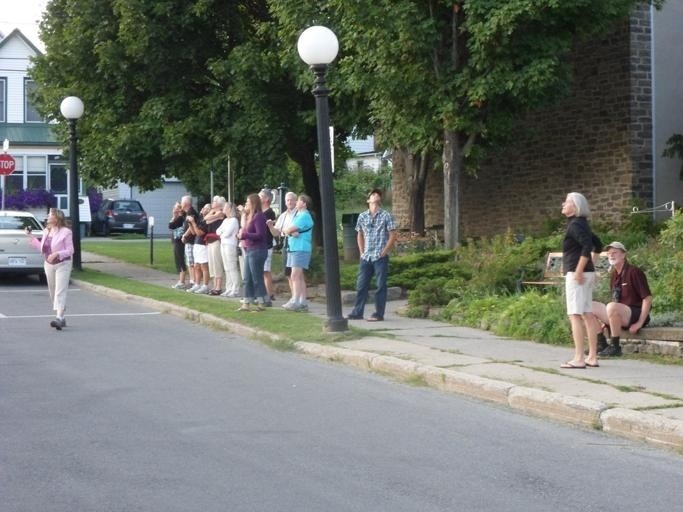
<box><xmin>343</xmin><ymin>189</ymin><xmax>397</xmax><ymax>322</ymax></box>
<box><xmin>583</xmin><ymin>241</ymin><xmax>653</xmax><ymax>357</ymax></box>
<box><xmin>24</xmin><ymin>208</ymin><xmax>75</xmax><ymax>331</ymax></box>
<box><xmin>561</xmin><ymin>192</ymin><xmax>602</xmax><ymax>368</ymax></box>
<box><xmin>168</xmin><ymin>189</ymin><xmax>315</xmax><ymax>312</ymax></box>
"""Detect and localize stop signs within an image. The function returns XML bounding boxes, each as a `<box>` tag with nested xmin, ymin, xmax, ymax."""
<box><xmin>0</xmin><ymin>152</ymin><xmax>17</xmax><ymax>177</ymax></box>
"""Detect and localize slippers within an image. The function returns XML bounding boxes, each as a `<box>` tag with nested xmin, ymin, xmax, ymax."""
<box><xmin>559</xmin><ymin>354</ymin><xmax>601</xmax><ymax>369</ymax></box>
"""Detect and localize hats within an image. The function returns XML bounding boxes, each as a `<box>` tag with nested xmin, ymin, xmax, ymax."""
<box><xmin>366</xmin><ymin>186</ymin><xmax>383</xmax><ymax>199</ymax></box>
<box><xmin>604</xmin><ymin>240</ymin><xmax>627</xmax><ymax>252</ymax></box>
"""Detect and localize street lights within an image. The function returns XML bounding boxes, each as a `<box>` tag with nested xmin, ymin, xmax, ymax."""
<box><xmin>59</xmin><ymin>95</ymin><xmax>84</xmax><ymax>271</ymax></box>
<box><xmin>295</xmin><ymin>22</ymin><xmax>348</xmax><ymax>334</ymax></box>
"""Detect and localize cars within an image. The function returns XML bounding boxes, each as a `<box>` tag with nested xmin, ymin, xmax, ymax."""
<box><xmin>0</xmin><ymin>207</ymin><xmax>48</xmax><ymax>284</ymax></box>
<box><xmin>91</xmin><ymin>198</ymin><xmax>149</xmax><ymax>238</ymax></box>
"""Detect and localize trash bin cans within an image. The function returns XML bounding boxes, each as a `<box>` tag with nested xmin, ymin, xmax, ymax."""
<box><xmin>342</xmin><ymin>213</ymin><xmax>360</xmax><ymax>260</ymax></box>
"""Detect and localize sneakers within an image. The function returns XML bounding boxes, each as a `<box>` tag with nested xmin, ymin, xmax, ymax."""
<box><xmin>204</xmin><ymin>288</ymin><xmax>223</xmax><ymax>296</ymax></box>
<box><xmin>225</xmin><ymin>291</ymin><xmax>241</xmax><ymax>298</ymax></box>
<box><xmin>49</xmin><ymin>316</ymin><xmax>67</xmax><ymax>331</ymax></box>
<box><xmin>170</xmin><ymin>280</ymin><xmax>193</xmax><ymax>290</ymax></box>
<box><xmin>193</xmin><ymin>284</ymin><xmax>209</xmax><ymax>295</ymax></box>
<box><xmin>597</xmin><ymin>344</ymin><xmax>622</xmax><ymax>357</ymax></box>
<box><xmin>366</xmin><ymin>315</ymin><xmax>384</xmax><ymax>322</ymax></box>
<box><xmin>219</xmin><ymin>289</ymin><xmax>232</xmax><ymax>297</ymax></box>
<box><xmin>184</xmin><ymin>283</ymin><xmax>201</xmax><ymax>293</ymax></box>
<box><xmin>583</xmin><ymin>342</ymin><xmax>608</xmax><ymax>355</ymax></box>
<box><xmin>234</xmin><ymin>292</ymin><xmax>277</xmax><ymax>313</ymax></box>
<box><xmin>280</xmin><ymin>300</ymin><xmax>309</xmax><ymax>312</ymax></box>
<box><xmin>343</xmin><ymin>315</ymin><xmax>364</xmax><ymax>320</ymax></box>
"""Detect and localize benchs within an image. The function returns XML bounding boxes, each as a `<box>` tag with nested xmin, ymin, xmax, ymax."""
<box><xmin>517</xmin><ymin>246</ymin><xmax>615</xmax><ymax>287</ymax></box>
